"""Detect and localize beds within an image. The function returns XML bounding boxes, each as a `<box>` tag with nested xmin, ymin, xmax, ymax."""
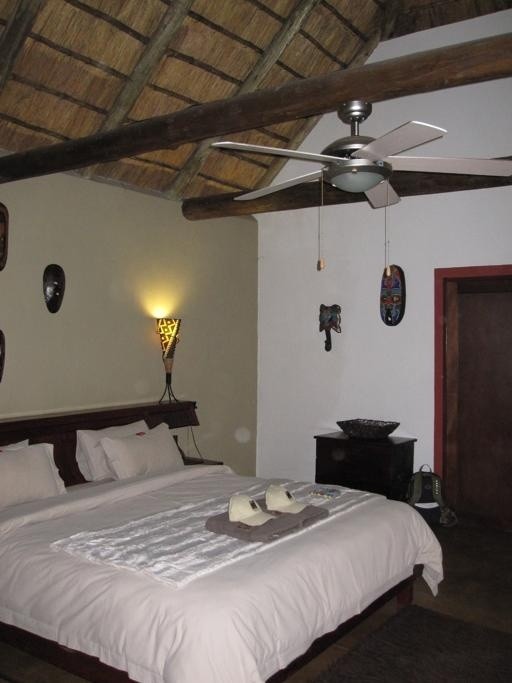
<box><xmin>0</xmin><ymin>399</ymin><xmax>416</xmax><ymax>682</ymax></box>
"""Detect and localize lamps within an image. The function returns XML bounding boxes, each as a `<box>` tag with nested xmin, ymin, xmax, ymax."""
<box><xmin>154</xmin><ymin>317</ymin><xmax>182</xmax><ymax>404</ymax></box>
<box><xmin>316</xmin><ymin>158</ymin><xmax>392</xmax><ymax>279</ymax></box>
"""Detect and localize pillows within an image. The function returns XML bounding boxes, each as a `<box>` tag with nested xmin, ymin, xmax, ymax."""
<box><xmin>0</xmin><ymin>437</ymin><xmax>69</xmax><ymax>507</ymax></box>
<box><xmin>75</xmin><ymin>418</ymin><xmax>185</xmax><ymax>481</ymax></box>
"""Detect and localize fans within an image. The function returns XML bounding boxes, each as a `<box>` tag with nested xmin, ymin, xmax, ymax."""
<box><xmin>209</xmin><ymin>99</ymin><xmax>511</xmax><ymax>209</ymax></box>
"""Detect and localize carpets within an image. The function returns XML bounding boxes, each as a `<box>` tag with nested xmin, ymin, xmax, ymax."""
<box><xmin>307</xmin><ymin>604</ymin><xmax>510</xmax><ymax>683</ymax></box>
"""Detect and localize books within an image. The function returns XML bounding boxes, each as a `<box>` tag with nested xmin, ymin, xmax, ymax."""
<box><xmin>311</xmin><ymin>486</ymin><xmax>340</xmax><ymax>499</ymax></box>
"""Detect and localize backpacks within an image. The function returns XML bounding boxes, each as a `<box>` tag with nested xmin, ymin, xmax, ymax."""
<box><xmin>403</xmin><ymin>463</ymin><xmax>459</xmax><ymax>528</ymax></box>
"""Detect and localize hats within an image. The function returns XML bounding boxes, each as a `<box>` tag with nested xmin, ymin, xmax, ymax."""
<box><xmin>228</xmin><ymin>493</ymin><xmax>276</xmax><ymax>527</ymax></box>
<box><xmin>265</xmin><ymin>484</ymin><xmax>309</xmax><ymax>514</ymax></box>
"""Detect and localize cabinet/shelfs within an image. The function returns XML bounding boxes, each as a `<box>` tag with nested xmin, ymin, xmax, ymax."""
<box><xmin>314</xmin><ymin>431</ymin><xmax>417</xmax><ymax>501</ymax></box>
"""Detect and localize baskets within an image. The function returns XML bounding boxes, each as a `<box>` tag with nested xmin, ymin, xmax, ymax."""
<box><xmin>336</xmin><ymin>418</ymin><xmax>400</xmax><ymax>439</ymax></box>
